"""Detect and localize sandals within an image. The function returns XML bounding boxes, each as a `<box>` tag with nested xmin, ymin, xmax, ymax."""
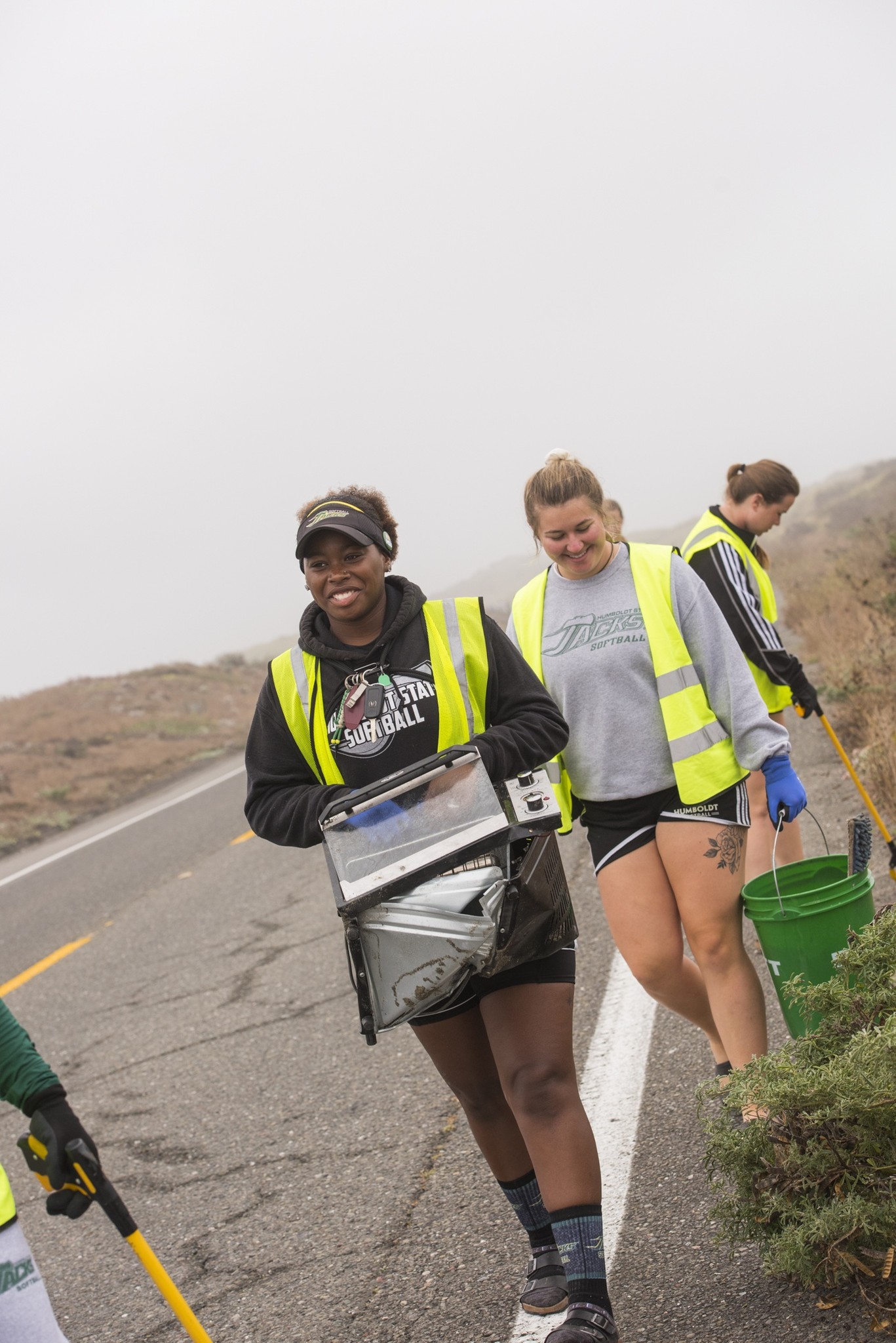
<box><xmin>541</xmin><ymin>1302</ymin><xmax>621</xmax><ymax>1343</ymax></box>
<box><xmin>519</xmin><ymin>1250</ymin><xmax>570</xmax><ymax>1316</ymax></box>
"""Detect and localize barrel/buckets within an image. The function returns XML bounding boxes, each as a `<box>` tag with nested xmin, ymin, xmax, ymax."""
<box><xmin>741</xmin><ymin>806</ymin><xmax>880</xmax><ymax>1042</ymax></box>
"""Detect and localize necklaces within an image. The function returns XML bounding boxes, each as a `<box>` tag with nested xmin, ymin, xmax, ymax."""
<box><xmin>557</xmin><ymin>539</ymin><xmax>613</xmax><ymax>577</ymax></box>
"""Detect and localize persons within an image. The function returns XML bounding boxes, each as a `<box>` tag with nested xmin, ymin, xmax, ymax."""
<box><xmin>244</xmin><ymin>489</ymin><xmax>621</xmax><ymax>1342</ymax></box>
<box><xmin>1</xmin><ymin>994</ymin><xmax>101</xmax><ymax>1343</ymax></box>
<box><xmin>674</xmin><ymin>455</ymin><xmax>825</xmax><ymax>877</ymax></box>
<box><xmin>601</xmin><ymin>498</ymin><xmax>627</xmax><ymax>544</ymax></box>
<box><xmin>502</xmin><ymin>444</ymin><xmax>809</xmax><ymax>1120</ymax></box>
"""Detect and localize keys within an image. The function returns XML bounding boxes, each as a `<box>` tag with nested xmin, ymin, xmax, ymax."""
<box><xmin>363</xmin><ymin>684</ymin><xmax>384</xmax><ymax>743</ymax></box>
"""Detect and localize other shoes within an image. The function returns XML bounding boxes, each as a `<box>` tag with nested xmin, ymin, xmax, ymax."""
<box><xmin>712</xmin><ymin>1059</ymin><xmax>742</xmax><ymax>1128</ymax></box>
<box><xmin>739</xmin><ymin>1101</ymin><xmax>783</xmax><ymax>1132</ymax></box>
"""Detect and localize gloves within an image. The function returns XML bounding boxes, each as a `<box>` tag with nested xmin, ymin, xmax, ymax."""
<box><xmin>17</xmin><ymin>1085</ymin><xmax>103</xmax><ymax>1221</ymax></box>
<box><xmin>760</xmin><ymin>754</ymin><xmax>810</xmax><ymax>832</ymax></box>
<box><xmin>789</xmin><ymin>672</ymin><xmax>825</xmax><ymax>718</ymax></box>
<box><xmin>337</xmin><ymin>791</ymin><xmax>412</xmax><ymax>848</ymax></box>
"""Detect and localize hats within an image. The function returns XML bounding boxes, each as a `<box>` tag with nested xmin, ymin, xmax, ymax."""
<box><xmin>295</xmin><ymin>502</ymin><xmax>395</xmax><ymax>573</ymax></box>
